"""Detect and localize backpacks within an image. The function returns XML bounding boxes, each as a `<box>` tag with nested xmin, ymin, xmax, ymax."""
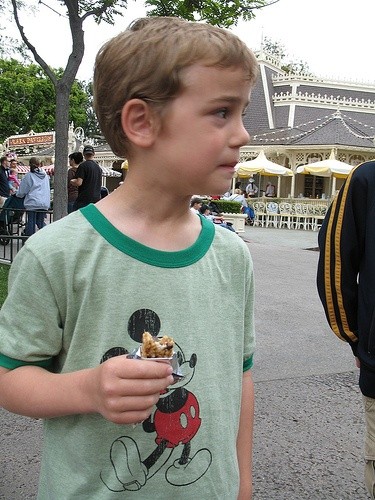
<box><xmin>242</xmin><ymin>205</ymin><xmax>254</xmax><ymax>218</ymax></box>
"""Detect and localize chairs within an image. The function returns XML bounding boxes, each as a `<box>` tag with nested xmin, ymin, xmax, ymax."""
<box><xmin>252</xmin><ymin>202</ymin><xmax>328</xmax><ymax>231</ymax></box>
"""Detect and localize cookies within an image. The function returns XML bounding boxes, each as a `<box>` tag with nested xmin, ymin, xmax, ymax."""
<box><xmin>141</xmin><ymin>332</ymin><xmax>174</xmax><ymax>358</ymax></box>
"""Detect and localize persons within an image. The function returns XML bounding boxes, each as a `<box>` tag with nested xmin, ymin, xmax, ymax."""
<box><xmin>316</xmin><ymin>158</ymin><xmax>375</xmax><ymax>500</ymax></box>
<box><xmin>0</xmin><ymin>145</ymin><xmax>276</xmax><ymax>236</ymax></box>
<box><xmin>0</xmin><ymin>17</ymin><xmax>257</xmax><ymax>500</ymax></box>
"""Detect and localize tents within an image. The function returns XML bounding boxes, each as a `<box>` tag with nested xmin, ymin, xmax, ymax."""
<box><xmin>232</xmin><ymin>150</ymin><xmax>294</xmax><ymax>199</ymax></box>
<box><xmin>295</xmin><ymin>150</ymin><xmax>355</xmax><ymax>200</ymax></box>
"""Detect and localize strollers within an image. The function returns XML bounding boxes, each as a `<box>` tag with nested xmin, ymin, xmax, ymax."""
<box><xmin>0</xmin><ymin>194</ymin><xmax>26</xmax><ymax>245</ymax></box>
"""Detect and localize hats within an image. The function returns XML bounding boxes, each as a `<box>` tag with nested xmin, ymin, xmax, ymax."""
<box><xmin>84</xmin><ymin>145</ymin><xmax>95</xmax><ymax>154</ymax></box>
<box><xmin>249</xmin><ymin>178</ymin><xmax>255</xmax><ymax>182</ymax></box>
<box><xmin>201</xmin><ymin>204</ymin><xmax>212</xmax><ymax>213</ymax></box>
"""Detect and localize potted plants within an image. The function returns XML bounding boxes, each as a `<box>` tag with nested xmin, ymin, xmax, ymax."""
<box><xmin>203</xmin><ymin>200</ymin><xmax>248</xmax><ymax>232</ymax></box>
